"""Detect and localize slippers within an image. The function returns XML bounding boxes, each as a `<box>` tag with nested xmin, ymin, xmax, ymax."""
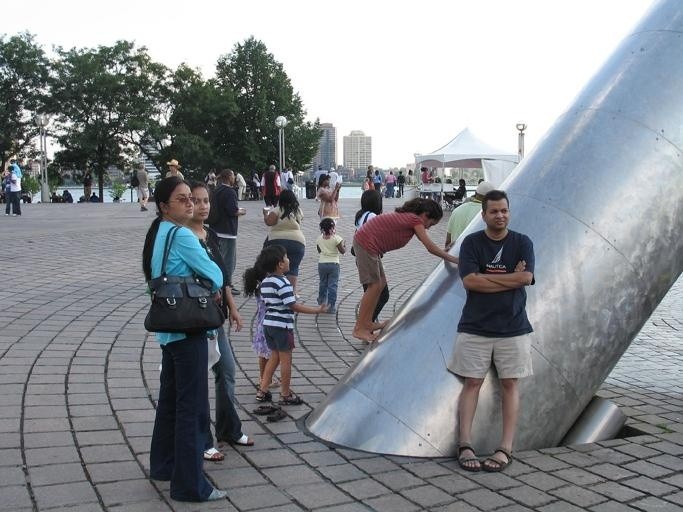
<box><xmin>253</xmin><ymin>405</ymin><xmax>281</xmax><ymax>415</ymax></box>
<box><xmin>266</xmin><ymin>408</ymin><xmax>290</xmax><ymax>421</ymax></box>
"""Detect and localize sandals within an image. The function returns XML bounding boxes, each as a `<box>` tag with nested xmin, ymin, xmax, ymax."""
<box><xmin>255</xmin><ymin>390</ymin><xmax>275</xmax><ymax>402</ymax></box>
<box><xmin>278</xmin><ymin>391</ymin><xmax>304</xmax><ymax>406</ymax></box>
<box><xmin>482</xmin><ymin>447</ymin><xmax>513</xmax><ymax>473</ymax></box>
<box><xmin>257</xmin><ymin>377</ymin><xmax>262</xmax><ymax>388</ymax></box>
<box><xmin>260</xmin><ymin>375</ymin><xmax>281</xmax><ymax>386</ymax></box>
<box><xmin>202</xmin><ymin>447</ymin><xmax>225</xmax><ymax>462</ymax></box>
<box><xmin>455</xmin><ymin>442</ymin><xmax>482</xmax><ymax>471</ymax></box>
<box><xmin>215</xmin><ymin>433</ymin><xmax>254</xmax><ymax>447</ymax></box>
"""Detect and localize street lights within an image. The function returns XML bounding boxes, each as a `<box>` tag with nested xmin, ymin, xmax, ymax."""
<box><xmin>38</xmin><ymin>115</ymin><xmax>50</xmax><ymax>202</ymax></box>
<box><xmin>516</xmin><ymin>120</ymin><xmax>528</xmax><ymax>163</ymax></box>
<box><xmin>275</xmin><ymin>116</ymin><xmax>287</xmax><ymax>170</ymax></box>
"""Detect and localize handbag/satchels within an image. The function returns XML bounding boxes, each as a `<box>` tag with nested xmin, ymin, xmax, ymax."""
<box><xmin>351</xmin><ymin>211</ymin><xmax>384</xmax><ymax>259</ymax></box>
<box><xmin>143</xmin><ymin>224</ymin><xmax>226</xmax><ymax>335</ymax></box>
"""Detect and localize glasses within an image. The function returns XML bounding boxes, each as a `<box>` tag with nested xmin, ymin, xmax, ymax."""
<box><xmin>164</xmin><ymin>195</ymin><xmax>196</xmax><ymax>204</ymax></box>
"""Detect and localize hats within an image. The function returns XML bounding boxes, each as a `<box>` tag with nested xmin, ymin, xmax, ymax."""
<box><xmin>475</xmin><ymin>180</ymin><xmax>495</xmax><ymax>196</ymax></box>
<box><xmin>165</xmin><ymin>159</ymin><xmax>182</xmax><ymax>169</ymax></box>
<box><xmin>329</xmin><ymin>167</ymin><xmax>336</xmax><ymax>172</ymax></box>
<box><xmin>318</xmin><ymin>174</ymin><xmax>331</xmax><ymax>186</ymax></box>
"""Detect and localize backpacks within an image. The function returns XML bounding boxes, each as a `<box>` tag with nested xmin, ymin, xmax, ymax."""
<box><xmin>130</xmin><ymin>170</ymin><xmax>140</xmax><ymax>187</ymax></box>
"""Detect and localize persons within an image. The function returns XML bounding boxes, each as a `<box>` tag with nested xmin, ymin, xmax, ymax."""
<box><xmin>262</xmin><ymin>190</ymin><xmax>306</xmax><ymax>300</ymax></box>
<box><xmin>1</xmin><ymin>159</ymin><xmax>22</xmax><ymax>216</ymax></box>
<box><xmin>235</xmin><ymin>170</ymin><xmax>246</xmax><ymax>201</ymax></box>
<box><xmin>204</xmin><ymin>169</ymin><xmax>246</xmax><ymax>295</ymax></box>
<box><xmin>316</xmin><ymin>218</ymin><xmax>345</xmax><ymax>313</ymax></box>
<box><xmin>185</xmin><ymin>180</ymin><xmax>254</xmax><ymax>461</ymax></box>
<box><xmin>420</xmin><ymin>168</ymin><xmax>467</xmax><ymax>210</ymax></box>
<box><xmin>50</xmin><ymin>189</ymin><xmax>73</xmax><ymax>203</ymax></box>
<box><xmin>396</xmin><ymin>170</ymin><xmax>405</xmax><ymax>196</ymax></box>
<box><xmin>166</xmin><ymin>159</ymin><xmax>184</xmax><ymax>180</ymax></box>
<box><xmin>261</xmin><ymin>165</ymin><xmax>282</xmax><ymax>207</ymax></box>
<box><xmin>444</xmin><ymin>181</ymin><xmax>497</xmax><ymax>253</ymax></box>
<box><xmin>142</xmin><ymin>177</ymin><xmax>227</xmax><ymax>503</ymax></box>
<box><xmin>318</xmin><ymin>174</ymin><xmax>342</xmax><ymax>226</ymax></box>
<box><xmin>205</xmin><ymin>167</ymin><xmax>217</xmax><ymax>201</ymax></box>
<box><xmin>373</xmin><ymin>170</ymin><xmax>381</xmax><ymax>192</ymax></box>
<box><xmin>314</xmin><ymin>165</ymin><xmax>329</xmax><ymax>201</ymax></box>
<box><xmin>328</xmin><ymin>167</ymin><xmax>338</xmax><ymax>188</ymax></box>
<box><xmin>89</xmin><ymin>192</ymin><xmax>99</xmax><ymax>202</ymax></box>
<box><xmin>385</xmin><ymin>171</ymin><xmax>397</xmax><ymax>198</ymax></box>
<box><xmin>447</xmin><ymin>191</ymin><xmax>538</xmax><ymax>474</ymax></box>
<box><xmin>243</xmin><ymin>262</ymin><xmax>270</xmax><ymax>388</ymax></box>
<box><xmin>131</xmin><ymin>164</ymin><xmax>149</xmax><ymax>212</ymax></box>
<box><xmin>279</xmin><ymin>166</ymin><xmax>299</xmax><ymax>195</ymax></box>
<box><xmin>406</xmin><ymin>170</ymin><xmax>416</xmax><ymax>186</ymax></box>
<box><xmin>361</xmin><ymin>165</ymin><xmax>374</xmax><ymax>192</ymax></box>
<box><xmin>353</xmin><ymin>197</ymin><xmax>460</xmax><ymax>341</ymax></box>
<box><xmin>256</xmin><ymin>245</ymin><xmax>331</xmax><ymax>406</ymax></box>
<box><xmin>351</xmin><ymin>190</ymin><xmax>390</xmax><ymax>336</ymax></box>
<box><xmin>253</xmin><ymin>172</ymin><xmax>260</xmax><ymax>200</ymax></box>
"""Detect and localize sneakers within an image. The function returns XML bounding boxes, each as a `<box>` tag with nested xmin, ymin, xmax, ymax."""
<box><xmin>230</xmin><ymin>288</ymin><xmax>241</xmax><ymax>295</ymax></box>
<box><xmin>141</xmin><ymin>207</ymin><xmax>148</xmax><ymax>211</ymax></box>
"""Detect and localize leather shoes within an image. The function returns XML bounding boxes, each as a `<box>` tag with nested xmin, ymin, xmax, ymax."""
<box><xmin>206</xmin><ymin>487</ymin><xmax>228</xmax><ymax>501</ymax></box>
